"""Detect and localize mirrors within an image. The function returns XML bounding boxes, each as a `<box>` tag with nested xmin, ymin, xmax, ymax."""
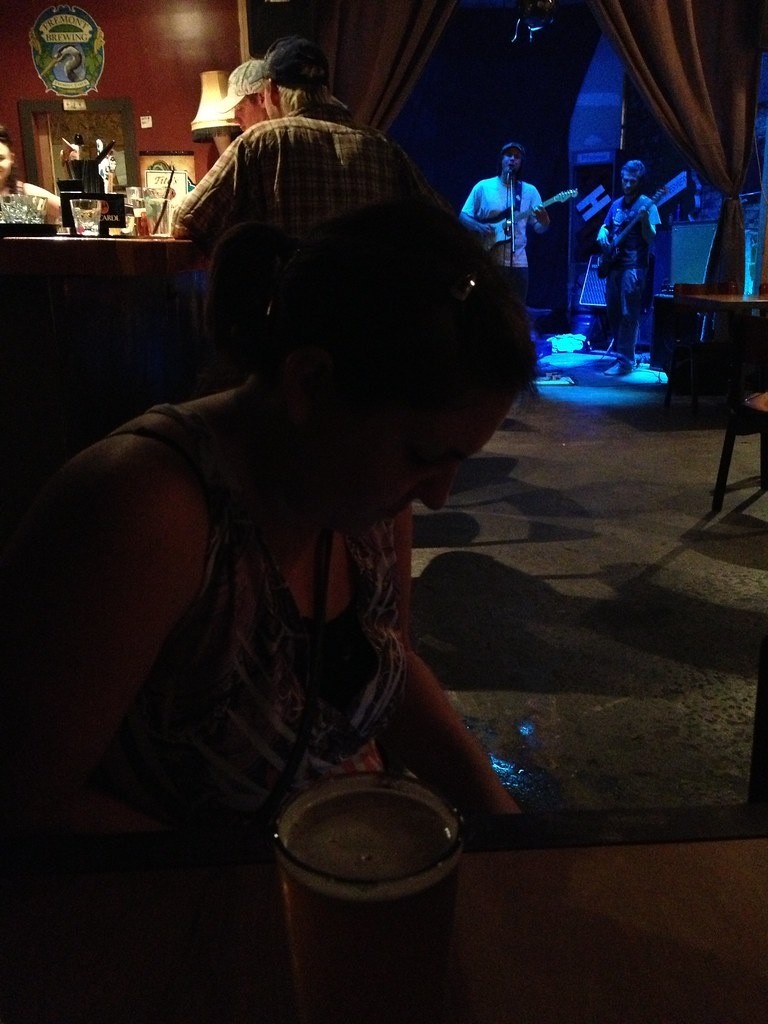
<box><xmin>18</xmin><ymin>98</ymin><xmax>138</xmax><ymax>194</ymax></box>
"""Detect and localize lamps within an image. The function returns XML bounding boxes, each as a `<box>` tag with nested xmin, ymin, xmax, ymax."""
<box><xmin>191</xmin><ymin>71</ymin><xmax>242</xmax><ymax>155</ymax></box>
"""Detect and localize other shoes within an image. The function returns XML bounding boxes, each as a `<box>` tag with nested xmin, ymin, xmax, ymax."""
<box><xmin>604</xmin><ymin>362</ymin><xmax>632</xmax><ymax>374</ymax></box>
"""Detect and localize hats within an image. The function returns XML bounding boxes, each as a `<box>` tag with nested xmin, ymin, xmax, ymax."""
<box><xmin>620</xmin><ymin>159</ymin><xmax>646</xmax><ymax>185</ymax></box>
<box><xmin>500</xmin><ymin>141</ymin><xmax>524</xmax><ymax>159</ymax></box>
<box><xmin>218</xmin><ymin>58</ymin><xmax>265</xmax><ymax>113</ymax></box>
<box><xmin>249</xmin><ymin>34</ymin><xmax>331</xmax><ymax>88</ymax></box>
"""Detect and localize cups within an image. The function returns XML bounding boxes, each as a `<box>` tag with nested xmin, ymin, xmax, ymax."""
<box><xmin>271</xmin><ymin>772</ymin><xmax>465</xmax><ymax>1024</ymax></box>
<box><xmin>0</xmin><ymin>194</ymin><xmax>49</xmax><ymax>225</ymax></box>
<box><xmin>69</xmin><ymin>198</ymin><xmax>102</xmax><ymax>236</ymax></box>
<box><xmin>142</xmin><ymin>187</ymin><xmax>172</xmax><ymax>237</ymax></box>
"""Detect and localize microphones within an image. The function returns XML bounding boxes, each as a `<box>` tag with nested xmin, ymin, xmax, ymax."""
<box><xmin>508</xmin><ymin>167</ymin><xmax>513</xmax><ymax>174</ymax></box>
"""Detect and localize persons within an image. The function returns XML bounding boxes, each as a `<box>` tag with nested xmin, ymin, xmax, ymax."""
<box><xmin>173</xmin><ymin>36</ymin><xmax>471</xmax><ymax>239</ymax></box>
<box><xmin>1</xmin><ymin>127</ymin><xmax>65</xmax><ymax>223</ymax></box>
<box><xmin>458</xmin><ymin>142</ymin><xmax>551</xmax><ymax>308</ymax></box>
<box><xmin>0</xmin><ymin>195</ymin><xmax>539</xmax><ymax>838</ymax></box>
<box><xmin>215</xmin><ymin>57</ymin><xmax>270</xmax><ymax>130</ymax></box>
<box><xmin>596</xmin><ymin>160</ymin><xmax>662</xmax><ymax>375</ymax></box>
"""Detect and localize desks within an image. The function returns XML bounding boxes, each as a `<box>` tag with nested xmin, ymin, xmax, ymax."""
<box><xmin>1</xmin><ymin>801</ymin><xmax>767</xmax><ymax>1024</ymax></box>
<box><xmin>2</xmin><ymin>234</ymin><xmax>214</xmax><ymax>444</ymax></box>
<box><xmin>680</xmin><ymin>294</ymin><xmax>768</xmax><ymax>415</ymax></box>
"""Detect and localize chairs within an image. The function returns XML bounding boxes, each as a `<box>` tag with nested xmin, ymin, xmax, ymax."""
<box><xmin>664</xmin><ymin>283</ymin><xmax>768</xmax><ymax>511</ymax></box>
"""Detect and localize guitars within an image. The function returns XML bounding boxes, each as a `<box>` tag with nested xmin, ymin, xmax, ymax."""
<box><xmin>480</xmin><ymin>187</ymin><xmax>581</xmax><ymax>248</ymax></box>
<box><xmin>598</xmin><ymin>184</ymin><xmax>671</xmax><ymax>280</ymax></box>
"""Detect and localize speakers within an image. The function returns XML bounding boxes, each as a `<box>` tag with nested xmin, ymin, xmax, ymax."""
<box><xmin>649</xmin><ymin>299</ymin><xmax>716</xmax><ymax>372</ymax></box>
<box><xmin>579</xmin><ymin>255</ymin><xmax>608</xmax><ymax>309</ymax></box>
<box><xmin>651</xmin><ymin>220</ymin><xmax>723</xmax><ymax>300</ymax></box>
